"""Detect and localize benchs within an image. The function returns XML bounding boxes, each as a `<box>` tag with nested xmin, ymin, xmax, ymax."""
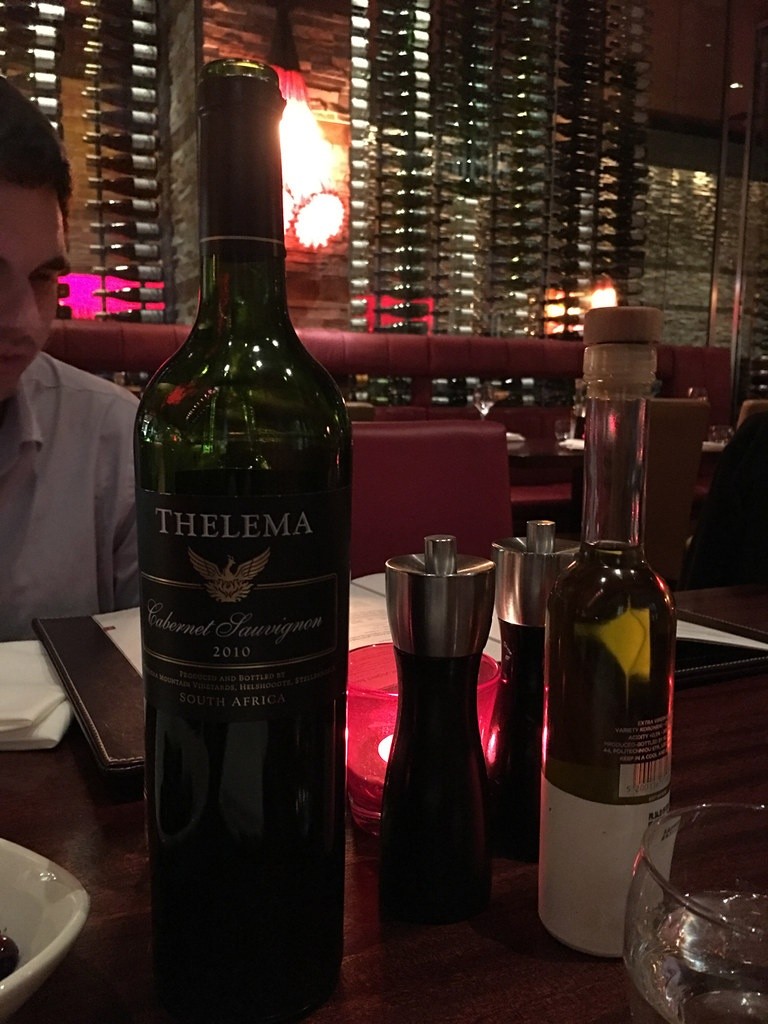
<box><xmin>321</xmin><ymin>405</ymin><xmax>584</xmax><ymax>580</ymax></box>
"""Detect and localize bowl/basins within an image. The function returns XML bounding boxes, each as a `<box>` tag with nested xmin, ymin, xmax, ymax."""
<box><xmin>0</xmin><ymin>838</ymin><xmax>91</xmax><ymax>1024</ymax></box>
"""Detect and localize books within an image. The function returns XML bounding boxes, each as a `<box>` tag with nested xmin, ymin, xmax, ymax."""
<box><xmin>32</xmin><ymin>572</ymin><xmax>768</xmax><ymax>771</ymax></box>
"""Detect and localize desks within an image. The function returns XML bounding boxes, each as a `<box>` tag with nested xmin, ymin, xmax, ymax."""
<box><xmin>509</xmin><ymin>439</ymin><xmax>583</xmax><ymax>541</ymax></box>
<box><xmin>0</xmin><ymin>586</ymin><xmax>768</xmax><ymax>1024</ymax></box>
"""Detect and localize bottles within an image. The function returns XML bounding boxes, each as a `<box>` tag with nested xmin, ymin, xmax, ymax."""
<box><xmin>0</xmin><ymin>0</ymin><xmax>177</xmax><ymax>385</ymax></box>
<box><xmin>515</xmin><ymin>309</ymin><xmax>678</xmax><ymax>958</ymax></box>
<box><xmin>350</xmin><ymin>0</ymin><xmax>650</xmax><ymax>406</ymax></box>
<box><xmin>131</xmin><ymin>56</ymin><xmax>354</xmax><ymax>1024</ymax></box>
<box><xmin>378</xmin><ymin>535</ymin><xmax>495</xmax><ymax>927</ymax></box>
<box><xmin>495</xmin><ymin>521</ymin><xmax>584</xmax><ymax>856</ymax></box>
<box><xmin>746</xmin><ymin>255</ymin><xmax>768</xmax><ymax>398</ymax></box>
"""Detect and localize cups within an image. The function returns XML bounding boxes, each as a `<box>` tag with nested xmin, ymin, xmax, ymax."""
<box><xmin>624</xmin><ymin>802</ymin><xmax>768</xmax><ymax>1024</ymax></box>
<box><xmin>346</xmin><ymin>642</ymin><xmax>505</xmax><ymax>837</ymax></box>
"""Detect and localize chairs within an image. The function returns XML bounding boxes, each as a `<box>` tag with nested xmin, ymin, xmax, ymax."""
<box><xmin>629</xmin><ymin>399</ymin><xmax>709</xmax><ymax>576</ymax></box>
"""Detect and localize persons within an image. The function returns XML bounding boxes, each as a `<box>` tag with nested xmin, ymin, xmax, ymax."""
<box><xmin>677</xmin><ymin>412</ymin><xmax>768</xmax><ymax>591</ymax></box>
<box><xmin>0</xmin><ymin>77</ymin><xmax>141</xmax><ymax>644</ymax></box>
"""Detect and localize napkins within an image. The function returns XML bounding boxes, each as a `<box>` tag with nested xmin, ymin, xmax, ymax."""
<box><xmin>0</xmin><ymin>638</ymin><xmax>73</xmax><ymax>751</ymax></box>
